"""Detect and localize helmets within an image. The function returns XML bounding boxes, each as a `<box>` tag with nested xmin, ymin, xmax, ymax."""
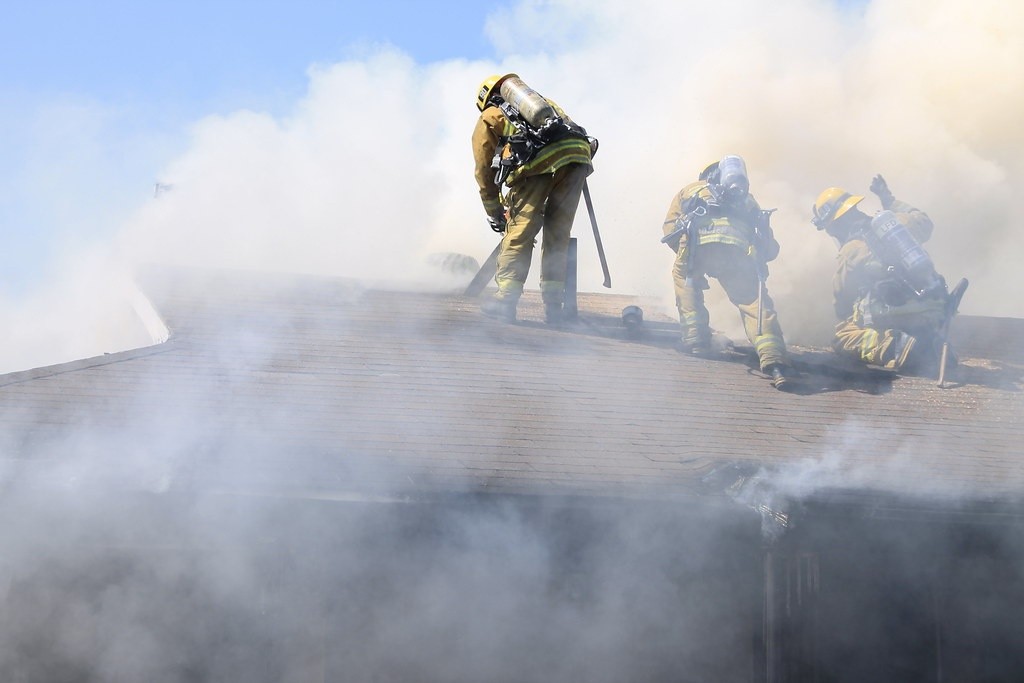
<box><xmin>698</xmin><ymin>160</ymin><xmax>720</xmax><ymax>180</ymax></box>
<box><xmin>475</xmin><ymin>73</ymin><xmax>521</xmax><ymax>112</ymax></box>
<box><xmin>811</xmin><ymin>187</ymin><xmax>865</xmax><ymax>230</ymax></box>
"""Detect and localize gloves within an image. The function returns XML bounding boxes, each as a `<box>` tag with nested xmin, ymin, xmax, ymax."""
<box><xmin>870</xmin><ymin>175</ymin><xmax>895</xmax><ymax>210</ymax></box>
<box><xmin>488</xmin><ymin>216</ymin><xmax>507</xmax><ymax>232</ymax></box>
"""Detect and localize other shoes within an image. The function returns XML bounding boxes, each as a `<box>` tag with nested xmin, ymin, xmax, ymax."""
<box><xmin>771</xmin><ymin>366</ymin><xmax>812</xmax><ymax>390</ymax></box>
<box><xmin>933</xmin><ymin>335</ymin><xmax>957</xmax><ymax>371</ymax></box>
<box><xmin>884</xmin><ymin>333</ymin><xmax>917</xmax><ymax>369</ymax></box>
<box><xmin>543</xmin><ymin>302</ymin><xmax>563</xmax><ymax>323</ymax></box>
<box><xmin>480</xmin><ymin>298</ymin><xmax>516</xmax><ymax>322</ymax></box>
<box><xmin>674</xmin><ymin>338</ymin><xmax>711</xmax><ymax>355</ymax></box>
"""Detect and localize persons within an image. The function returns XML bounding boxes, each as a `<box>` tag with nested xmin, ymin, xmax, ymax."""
<box><xmin>663</xmin><ymin>160</ymin><xmax>812</xmax><ymax>392</ymax></box>
<box><xmin>472</xmin><ymin>73</ymin><xmax>594</xmax><ymax>323</ymax></box>
<box><xmin>812</xmin><ymin>174</ymin><xmax>949</xmax><ymax>370</ymax></box>
